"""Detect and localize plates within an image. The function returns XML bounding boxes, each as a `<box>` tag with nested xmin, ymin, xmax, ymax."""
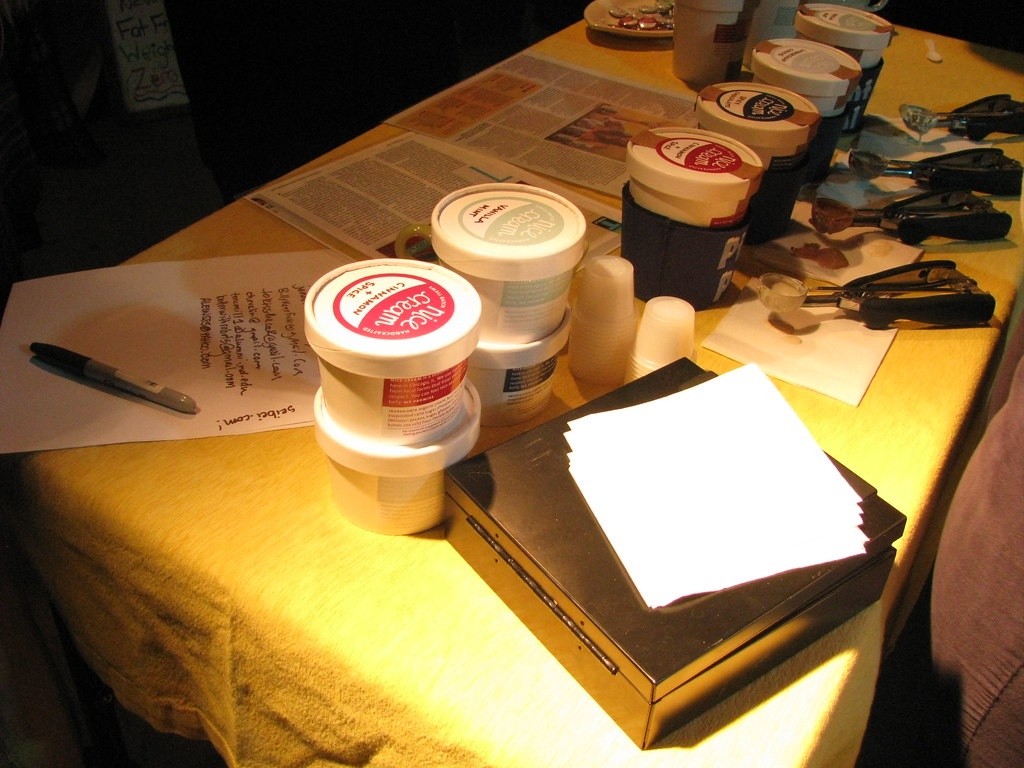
<box><xmin>583</xmin><ymin>0</ymin><xmax>676</xmax><ymax>39</ymax></box>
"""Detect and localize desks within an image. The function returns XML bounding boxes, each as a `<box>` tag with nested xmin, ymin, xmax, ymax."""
<box><xmin>1</xmin><ymin>5</ymin><xmax>1024</xmax><ymax>768</ymax></box>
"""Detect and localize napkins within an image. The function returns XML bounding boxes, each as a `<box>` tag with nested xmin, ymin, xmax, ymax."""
<box><xmin>813</xmin><ymin>109</ymin><xmax>994</xmax><ymax>221</ymax></box>
<box><xmin>704</xmin><ymin>274</ymin><xmax>899</xmax><ymax>406</ymax></box>
<box><xmin>756</xmin><ymin>199</ymin><xmax>924</xmax><ymax>292</ymax></box>
<box><xmin>563</xmin><ymin>362</ymin><xmax>870</xmax><ymax>610</ymax></box>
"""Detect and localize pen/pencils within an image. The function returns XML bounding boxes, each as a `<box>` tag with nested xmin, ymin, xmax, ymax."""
<box><xmin>28</xmin><ymin>341</ymin><xmax>197</xmax><ymax>414</ymax></box>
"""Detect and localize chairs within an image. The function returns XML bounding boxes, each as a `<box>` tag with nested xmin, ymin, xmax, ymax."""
<box><xmin>861</xmin><ymin>357</ymin><xmax>1024</xmax><ymax>768</ymax></box>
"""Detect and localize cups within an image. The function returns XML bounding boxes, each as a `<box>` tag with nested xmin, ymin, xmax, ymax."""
<box><xmin>568</xmin><ymin>256</ymin><xmax>697</xmax><ymax>389</ymax></box>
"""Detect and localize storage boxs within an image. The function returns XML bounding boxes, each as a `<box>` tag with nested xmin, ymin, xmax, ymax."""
<box><xmin>441</xmin><ymin>355</ymin><xmax>908</xmax><ymax>753</ymax></box>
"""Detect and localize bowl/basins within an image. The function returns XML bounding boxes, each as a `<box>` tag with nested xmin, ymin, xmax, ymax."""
<box><xmin>300</xmin><ymin>181</ymin><xmax>585</xmax><ymax>537</ymax></box>
<box><xmin>616</xmin><ymin>2</ymin><xmax>895</xmax><ymax>301</ymax></box>
